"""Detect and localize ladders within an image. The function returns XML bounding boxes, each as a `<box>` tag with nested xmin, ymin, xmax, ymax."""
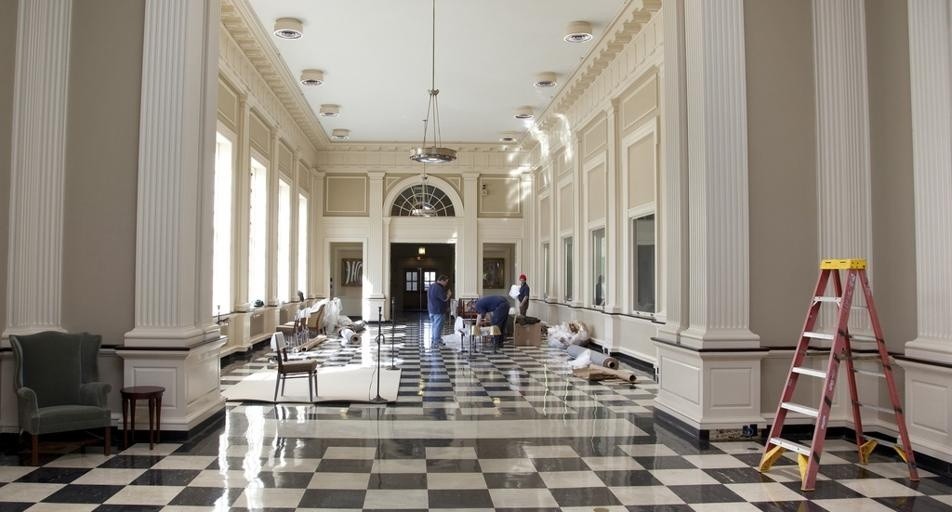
<box><xmin>757</xmin><ymin>259</ymin><xmax>920</xmax><ymax>491</ymax></box>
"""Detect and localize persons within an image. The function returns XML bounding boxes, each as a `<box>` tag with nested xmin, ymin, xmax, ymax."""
<box><xmin>424</xmin><ymin>272</ymin><xmax>453</xmax><ymax>350</ymax></box>
<box><xmin>425</xmin><ymin>352</ymin><xmax>445</xmax><ymax>412</ymax></box>
<box><xmin>516</xmin><ymin>274</ymin><xmax>531</xmax><ymax>317</ymax></box>
<box><xmin>465</xmin><ymin>296</ymin><xmax>511</xmax><ymax>348</ymax></box>
<box><xmin>596</xmin><ymin>272</ymin><xmax>605</xmax><ymax>304</ymax></box>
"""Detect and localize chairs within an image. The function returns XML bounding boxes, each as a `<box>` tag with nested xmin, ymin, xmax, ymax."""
<box><xmin>9</xmin><ymin>331</ymin><xmax>112</xmax><ymax>465</ymax></box>
<box><xmin>274</xmin><ymin>302</ymin><xmax>325</xmax><ymax>403</ymax></box>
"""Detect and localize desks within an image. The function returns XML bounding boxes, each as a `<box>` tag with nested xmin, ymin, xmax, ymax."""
<box><xmin>460</xmin><ymin>320</ymin><xmax>498</xmax><ymax>361</ymax></box>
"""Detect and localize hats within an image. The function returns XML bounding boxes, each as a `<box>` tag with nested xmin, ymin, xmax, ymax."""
<box><xmin>519</xmin><ymin>274</ymin><xmax>526</xmax><ymax>279</ymax></box>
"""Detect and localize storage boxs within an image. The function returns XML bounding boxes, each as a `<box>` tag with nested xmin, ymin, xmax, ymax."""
<box><xmin>513</xmin><ymin>315</ymin><xmax>542</xmax><ymax>346</ymax></box>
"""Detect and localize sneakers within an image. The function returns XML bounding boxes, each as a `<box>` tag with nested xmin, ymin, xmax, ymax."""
<box><xmin>431</xmin><ymin>342</ymin><xmax>446</xmax><ymax>349</ymax></box>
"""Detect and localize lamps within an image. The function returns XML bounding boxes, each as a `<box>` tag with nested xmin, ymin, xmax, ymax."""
<box><xmin>499</xmin><ymin>20</ymin><xmax>592</xmax><ymax>144</ymax></box>
<box><xmin>417</xmin><ymin>245</ymin><xmax>426</xmax><ymax>260</ymax></box>
<box><xmin>408</xmin><ymin>0</ymin><xmax>456</xmax><ymax>163</ymax></box>
<box><xmin>274</xmin><ymin>18</ymin><xmax>351</xmax><ymax>141</ymax></box>
<box><xmin>411</xmin><ymin>164</ymin><xmax>435</xmax><ymax>218</ymax></box>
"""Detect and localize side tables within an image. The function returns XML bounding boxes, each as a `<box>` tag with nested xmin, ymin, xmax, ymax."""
<box><xmin>120</xmin><ymin>385</ymin><xmax>166</xmax><ymax>451</ymax></box>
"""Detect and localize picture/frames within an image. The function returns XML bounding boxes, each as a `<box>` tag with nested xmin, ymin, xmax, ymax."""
<box><xmin>341</xmin><ymin>258</ymin><xmax>362</xmax><ymax>287</ymax></box>
<box><xmin>483</xmin><ymin>258</ymin><xmax>504</xmax><ymax>290</ymax></box>
